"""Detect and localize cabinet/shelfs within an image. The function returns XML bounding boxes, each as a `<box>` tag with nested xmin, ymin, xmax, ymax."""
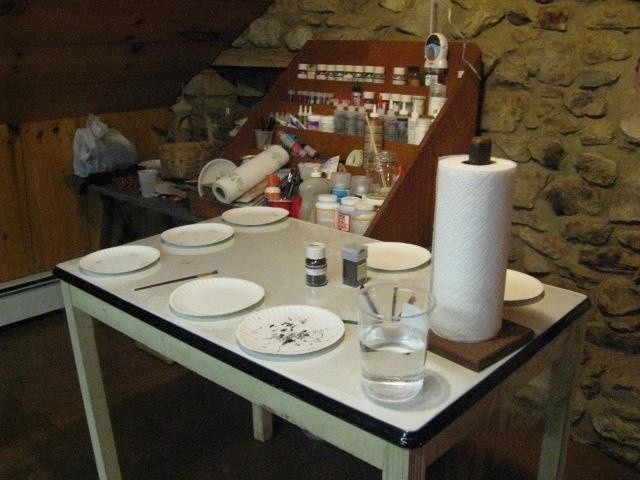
<box><xmin>201</xmin><ymin>34</ymin><xmax>483</xmax><ymax>252</ymax></box>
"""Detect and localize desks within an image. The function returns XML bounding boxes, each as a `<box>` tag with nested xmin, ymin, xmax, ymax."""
<box><xmin>51</xmin><ymin>205</ymin><xmax>594</xmax><ymax>480</ymax></box>
<box><xmin>65</xmin><ymin>164</ymin><xmax>200</xmax><ymax>252</ymax></box>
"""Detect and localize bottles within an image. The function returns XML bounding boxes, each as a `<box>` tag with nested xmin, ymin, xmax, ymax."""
<box><xmin>303</xmin><ymin>242</ymin><xmax>329</xmax><ymax>286</ymax></box>
<box><xmin>253</xmin><ymin>57</ymin><xmax>448</xmax><ymax>235</ymax></box>
<box><xmin>342</xmin><ymin>242</ymin><xmax>369</xmax><ymax>285</ymax></box>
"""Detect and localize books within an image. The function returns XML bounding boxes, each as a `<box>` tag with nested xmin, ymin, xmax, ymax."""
<box><xmin>233</xmin><ymin>167</ymin><xmax>300</xmax><ymax>208</ymax></box>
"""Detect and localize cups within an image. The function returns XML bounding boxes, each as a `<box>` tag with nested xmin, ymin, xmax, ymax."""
<box><xmin>356</xmin><ymin>284</ymin><xmax>436</xmax><ymax>405</ymax></box>
<box><xmin>136</xmin><ymin>169</ymin><xmax>162</xmax><ymax>198</ymax></box>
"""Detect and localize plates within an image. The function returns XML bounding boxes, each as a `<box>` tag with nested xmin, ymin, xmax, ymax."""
<box><xmin>161</xmin><ymin>234</ymin><xmax>239</xmax><ymax>256</ymax></box>
<box><xmin>80</xmin><ymin>244</ymin><xmax>160</xmax><ymax>275</ymax></box>
<box><xmin>230</xmin><ymin>216</ymin><xmax>292</xmax><ymax>235</ymax></box>
<box><xmin>363</xmin><ymin>240</ymin><xmax>431</xmax><ymax>272</ymax></box>
<box><xmin>236</xmin><ymin>305</ymin><xmax>344</xmax><ymax>357</ymax></box>
<box><xmin>80</xmin><ymin>259</ymin><xmax>164</xmax><ymax>282</ymax></box>
<box><xmin>196</xmin><ymin>157</ymin><xmax>238</xmax><ymax>198</ymax></box>
<box><xmin>166</xmin><ymin>295</ymin><xmax>264</xmax><ymax>326</ymax></box>
<box><xmin>234</xmin><ymin>335</ymin><xmax>344</xmax><ymax>363</ymax></box>
<box><xmin>169</xmin><ymin>278</ymin><xmax>263</xmax><ymax>317</ymax></box>
<box><xmin>159</xmin><ymin>222</ymin><xmax>235</xmax><ymax>247</ymax></box>
<box><xmin>222</xmin><ymin>206</ymin><xmax>289</xmax><ymax>226</ymax></box>
<box><xmin>502</xmin><ymin>268</ymin><xmax>545</xmax><ymax>303</ymax></box>
